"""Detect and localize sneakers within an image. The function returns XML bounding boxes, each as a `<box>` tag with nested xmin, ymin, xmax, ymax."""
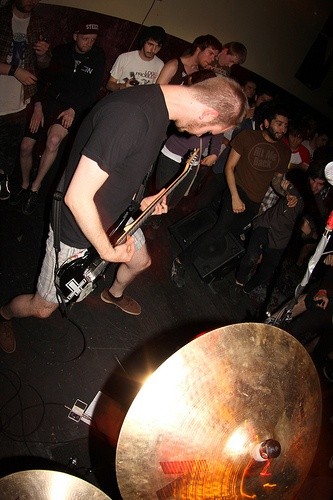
<box><xmin>0</xmin><ymin>307</ymin><xmax>17</xmax><ymax>354</ymax></box>
<box><xmin>235</xmin><ymin>268</ymin><xmax>257</xmax><ymax>285</ymax></box>
<box><xmin>241</xmin><ymin>281</ymin><xmax>267</xmax><ymax>298</ymax></box>
<box><xmin>172</xmin><ymin>256</ymin><xmax>188</xmax><ymax>289</ymax></box>
<box><xmin>0</xmin><ymin>172</ymin><xmax>11</xmax><ymax>200</ymax></box>
<box><xmin>22</xmin><ymin>184</ymin><xmax>43</xmax><ymax>215</ymax></box>
<box><xmin>9</xmin><ymin>183</ymin><xmax>28</xmax><ymax>206</ymax></box>
<box><xmin>100</xmin><ymin>288</ymin><xmax>142</xmax><ymax>316</ymax></box>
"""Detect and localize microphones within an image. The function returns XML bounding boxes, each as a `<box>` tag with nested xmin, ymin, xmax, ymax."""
<box><xmin>325</xmin><ymin>162</ymin><xmax>333</xmax><ymax>185</ymax></box>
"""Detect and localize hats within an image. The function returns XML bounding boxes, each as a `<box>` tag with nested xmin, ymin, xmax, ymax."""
<box><xmin>74</xmin><ymin>20</ymin><xmax>99</xmax><ymax>35</ymax></box>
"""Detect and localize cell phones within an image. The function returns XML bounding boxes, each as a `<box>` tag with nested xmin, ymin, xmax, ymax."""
<box><xmin>130</xmin><ymin>78</ymin><xmax>139</xmax><ymax>86</ymax></box>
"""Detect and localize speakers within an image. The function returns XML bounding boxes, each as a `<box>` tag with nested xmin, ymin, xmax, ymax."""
<box><xmin>169</xmin><ymin>206</ymin><xmax>245</xmax><ymax>282</ymax></box>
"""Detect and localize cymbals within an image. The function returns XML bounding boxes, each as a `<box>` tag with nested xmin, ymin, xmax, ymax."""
<box><xmin>115</xmin><ymin>323</ymin><xmax>325</xmax><ymax>500</ymax></box>
<box><xmin>0</xmin><ymin>469</ymin><xmax>111</xmax><ymax>500</ymax></box>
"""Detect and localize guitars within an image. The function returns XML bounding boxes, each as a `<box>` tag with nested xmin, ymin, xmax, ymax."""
<box><xmin>54</xmin><ymin>146</ymin><xmax>200</xmax><ymax>303</ymax></box>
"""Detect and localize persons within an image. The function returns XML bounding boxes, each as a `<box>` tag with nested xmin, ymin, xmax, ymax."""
<box><xmin>230</xmin><ymin>79</ymin><xmax>272</xmax><ymax>135</ymax></box>
<box><xmin>229</xmin><ymin>159</ymin><xmax>329</xmax><ymax>300</ymax></box>
<box><xmin>269</xmin><ymin>126</ymin><xmax>333</xmax><ymax>347</ymax></box>
<box><xmin>258</xmin><ymin>124</ymin><xmax>311</xmax><ymax>214</ymax></box>
<box><xmin>168</xmin><ymin>104</ymin><xmax>298</xmax><ymax>288</ymax></box>
<box><xmin>157</xmin><ymin>34</ymin><xmax>221</xmax><ymax>85</ymax></box>
<box><xmin>133</xmin><ymin>68</ymin><xmax>226</xmax><ymax>230</ymax></box>
<box><xmin>0</xmin><ymin>76</ymin><xmax>247</xmax><ymax>355</ymax></box>
<box><xmin>104</xmin><ymin>26</ymin><xmax>168</xmax><ymax>91</ymax></box>
<box><xmin>184</xmin><ymin>41</ymin><xmax>249</xmax><ymax>86</ymax></box>
<box><xmin>0</xmin><ymin>0</ymin><xmax>53</xmax><ymax>200</ymax></box>
<box><xmin>11</xmin><ymin>12</ymin><xmax>106</xmax><ymax>214</ymax></box>
